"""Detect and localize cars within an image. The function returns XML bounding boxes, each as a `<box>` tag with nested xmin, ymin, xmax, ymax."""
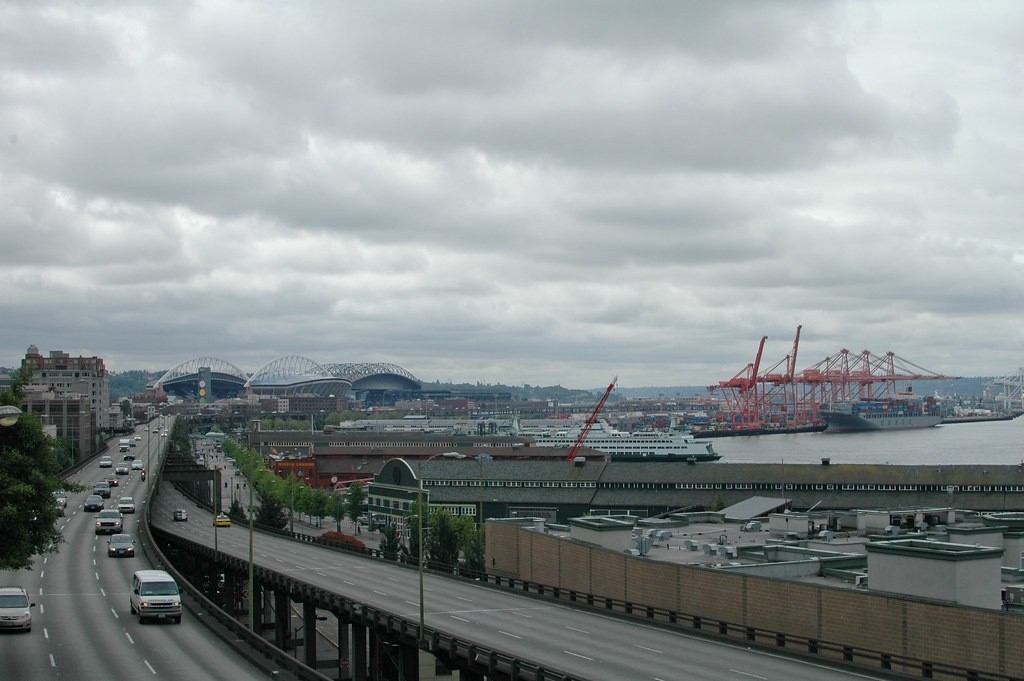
<box><xmin>118</xmin><ymin>497</ymin><xmax>136</xmax><ymax>513</ymax></box>
<box><xmin>99</xmin><ymin>456</ymin><xmax>113</xmax><ymax>468</ymax></box>
<box><xmin>83</xmin><ymin>495</ymin><xmax>105</xmax><ymax>512</ymax></box>
<box><xmin>212</xmin><ymin>514</ymin><xmax>232</xmax><ymax>528</ymax></box>
<box><xmin>115</xmin><ymin>413</ymin><xmax>171</xmax><ymax>475</ymax></box>
<box><xmin>51</xmin><ymin>491</ymin><xmax>69</xmax><ymax>515</ymax></box>
<box><xmin>174</xmin><ymin>508</ymin><xmax>189</xmax><ymax>521</ymax></box>
<box><xmin>107</xmin><ymin>533</ymin><xmax>136</xmax><ymax>558</ymax></box>
<box><xmin>104</xmin><ymin>473</ymin><xmax>119</xmax><ymax>486</ymax></box>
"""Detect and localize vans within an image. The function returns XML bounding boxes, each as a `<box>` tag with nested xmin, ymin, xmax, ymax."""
<box><xmin>130</xmin><ymin>569</ymin><xmax>184</xmax><ymax>623</ymax></box>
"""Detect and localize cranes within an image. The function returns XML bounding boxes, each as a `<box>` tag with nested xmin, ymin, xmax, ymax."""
<box><xmin>567</xmin><ymin>375</ymin><xmax>619</xmax><ymax>463</ymax></box>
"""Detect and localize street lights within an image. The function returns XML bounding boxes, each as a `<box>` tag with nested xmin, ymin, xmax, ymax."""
<box><xmin>213</xmin><ymin>457</ymin><xmax>238</xmax><ymax>564</ymax></box>
<box><xmin>248</xmin><ymin>470</ymin><xmax>274</xmax><ymax>631</ymax></box>
<box><xmin>416</xmin><ymin>450</ymin><xmax>466</xmax><ymax>644</ymax></box>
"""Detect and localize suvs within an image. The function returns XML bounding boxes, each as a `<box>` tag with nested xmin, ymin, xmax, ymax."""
<box><xmin>94</xmin><ymin>509</ymin><xmax>125</xmax><ymax>535</ymax></box>
<box><xmin>0</xmin><ymin>586</ymin><xmax>36</xmax><ymax>633</ymax></box>
<box><xmin>92</xmin><ymin>481</ymin><xmax>112</xmax><ymax>499</ymax></box>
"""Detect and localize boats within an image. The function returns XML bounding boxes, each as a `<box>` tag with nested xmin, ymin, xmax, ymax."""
<box><xmin>821</xmin><ymin>395</ymin><xmax>942</xmax><ymax>434</ymax></box>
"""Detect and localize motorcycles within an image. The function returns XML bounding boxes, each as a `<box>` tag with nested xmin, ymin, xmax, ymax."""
<box><xmin>141</xmin><ymin>473</ymin><xmax>145</xmax><ymax>481</ymax></box>
